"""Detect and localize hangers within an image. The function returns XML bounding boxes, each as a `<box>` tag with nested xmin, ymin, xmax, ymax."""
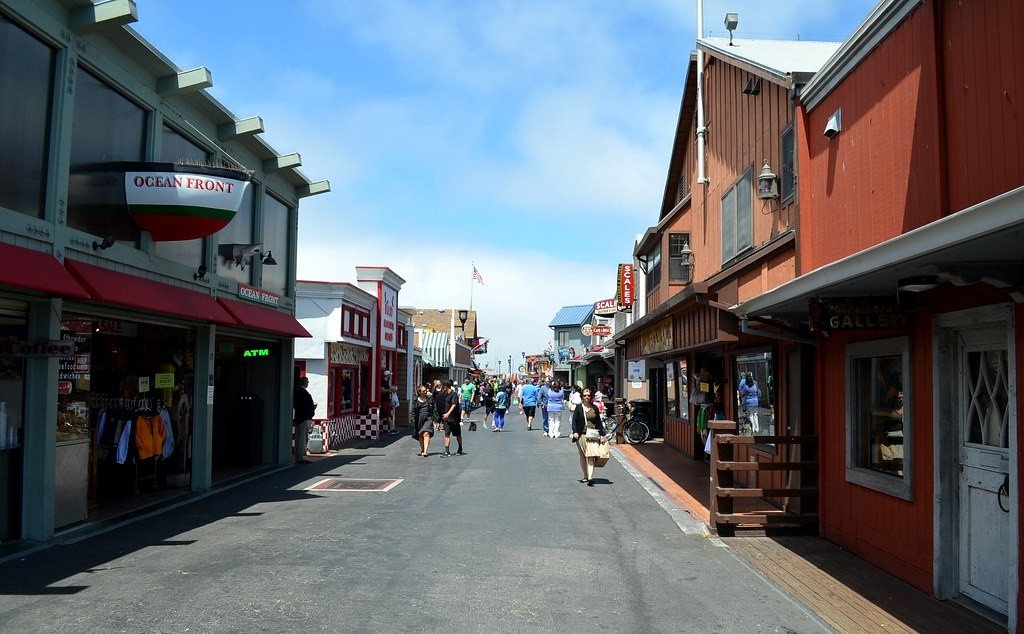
<box><xmin>88</xmin><ymin>395</ymin><xmax>166</xmax><ymax>419</ymax></box>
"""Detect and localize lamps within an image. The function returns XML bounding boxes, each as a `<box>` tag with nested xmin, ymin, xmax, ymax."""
<box><xmin>194</xmin><ymin>266</ymin><xmax>207</xmax><ymax>280</ymax></box>
<box><xmin>260</xmin><ymin>250</ymin><xmax>277</xmax><ymax>265</ymax></box>
<box><xmin>680</xmin><ymin>241</ymin><xmax>695</xmax><ymax>268</ymax></box>
<box><xmin>724</xmin><ymin>13</ymin><xmax>738</xmax><ymax>45</ymax></box>
<box><xmin>756</xmin><ymin>159</ymin><xmax>780</xmax><ymax>199</ymax></box>
<box><xmin>92</xmin><ymin>237</ymin><xmax>115</xmax><ymax>251</ymax></box>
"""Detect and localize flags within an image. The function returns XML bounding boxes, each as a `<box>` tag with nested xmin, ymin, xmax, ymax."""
<box><xmin>473</xmin><ymin>267</ymin><xmax>484</xmax><ymax>285</ymax></box>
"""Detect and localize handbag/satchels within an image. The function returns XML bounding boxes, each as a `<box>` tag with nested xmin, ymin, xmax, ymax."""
<box><xmin>571</xmin><ymin>403</ymin><xmax>577</xmax><ymax>412</ymax></box>
<box><xmin>391</xmin><ymin>391</ymin><xmax>399</xmax><ymax>408</ymax></box>
<box><xmin>586</xmin><ymin>428</ymin><xmax>600</xmax><ymax>440</ymax></box>
<box><xmin>513</xmin><ymin>399</ymin><xmax>518</xmax><ymax>406</ymax></box>
<box><xmin>594</xmin><ymin>442</ymin><xmax>610</xmax><ymax>467</ymax></box>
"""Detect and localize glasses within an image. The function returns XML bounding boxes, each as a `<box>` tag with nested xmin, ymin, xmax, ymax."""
<box><xmin>583</xmin><ymin>395</ymin><xmax>591</xmax><ymax>397</ymax></box>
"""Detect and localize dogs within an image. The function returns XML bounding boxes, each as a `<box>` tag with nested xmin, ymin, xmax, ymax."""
<box><xmin>467</xmin><ymin>422</ymin><xmax>477</xmax><ymax>432</ymax></box>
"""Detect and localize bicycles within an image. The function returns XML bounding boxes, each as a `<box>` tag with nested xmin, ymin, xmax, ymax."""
<box><xmin>601</xmin><ymin>402</ymin><xmax>650</xmax><ymax>445</ymax></box>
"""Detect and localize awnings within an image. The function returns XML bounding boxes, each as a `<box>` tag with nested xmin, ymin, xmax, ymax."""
<box><xmin>0</xmin><ymin>242</ymin><xmax>314</xmax><ymax>338</ymax></box>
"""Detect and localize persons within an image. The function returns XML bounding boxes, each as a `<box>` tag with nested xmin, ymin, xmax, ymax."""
<box><xmin>547</xmin><ymin>382</ymin><xmax>564</xmax><ymax>438</ymax></box>
<box><xmin>739</xmin><ymin>370</ymin><xmax>774</xmax><ymax>433</ymax></box>
<box><xmin>571</xmin><ymin>388</ymin><xmax>606</xmax><ymax>485</ymax></box>
<box><xmin>381</xmin><ymin>371</ymin><xmax>399</xmax><ymax>434</ymax></box>
<box><xmin>472</xmin><ymin>378</ymin><xmax>516</xmax><ymax>413</ymax></box>
<box><xmin>492</xmin><ymin>387</ymin><xmax>509</xmax><ymax>432</ymax></box>
<box><xmin>514</xmin><ymin>380</ymin><xmax>525</xmax><ymax>415</ymax></box>
<box><xmin>536</xmin><ymin>380</ymin><xmax>552</xmax><ymax>437</ymax></box>
<box><xmin>293</xmin><ymin>376</ymin><xmax>317</xmax><ymax>464</ymax></box>
<box><xmin>483</xmin><ymin>383</ymin><xmax>497</xmax><ymax>428</ymax></box>
<box><xmin>518</xmin><ymin>378</ymin><xmax>539</xmax><ymax>431</ymax></box>
<box><xmin>440</xmin><ymin>380</ymin><xmax>462</xmax><ymax>458</ymax></box>
<box><xmin>409</xmin><ymin>385</ymin><xmax>435</xmax><ymax>456</ymax></box>
<box><xmin>532</xmin><ymin>378</ymin><xmax>571</xmax><ymax>409</ymax></box>
<box><xmin>460</xmin><ymin>377</ymin><xmax>476</xmax><ymax>426</ymax></box>
<box><xmin>569</xmin><ymin>384</ymin><xmax>581</xmax><ymax>438</ymax></box>
<box><xmin>426</xmin><ymin>379</ymin><xmax>461</xmax><ymax>431</ymax></box>
<box><xmin>881</xmin><ymin>388</ymin><xmax>903</xmax><ymax>473</ymax></box>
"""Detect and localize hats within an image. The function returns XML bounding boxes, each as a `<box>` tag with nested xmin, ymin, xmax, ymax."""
<box><xmin>383</xmin><ymin>371</ymin><xmax>393</xmax><ymax>376</ymax></box>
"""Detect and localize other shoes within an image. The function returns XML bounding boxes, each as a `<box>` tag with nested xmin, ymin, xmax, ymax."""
<box><xmin>456</xmin><ymin>451</ymin><xmax>462</xmax><ymax>456</ymax></box>
<box><xmin>589</xmin><ymin>479</ymin><xmax>595</xmax><ymax>486</ymax></box>
<box><xmin>492</xmin><ymin>428</ymin><xmax>502</xmax><ymax>432</ymax></box>
<box><xmin>527</xmin><ymin>424</ymin><xmax>532</xmax><ymax>431</ymax></box>
<box><xmin>483</xmin><ymin>421</ymin><xmax>487</xmax><ymax>429</ymax></box>
<box><xmin>441</xmin><ymin>452</ymin><xmax>450</xmax><ymax>458</ymax></box>
<box><xmin>295</xmin><ymin>459</ymin><xmax>312</xmax><ymax>464</ymax></box>
<box><xmin>420</xmin><ymin>451</ymin><xmax>428</xmax><ymax>456</ymax></box>
<box><xmin>436</xmin><ymin>428</ymin><xmax>445</xmax><ymax>431</ymax></box>
<box><xmin>544</xmin><ymin>432</ymin><xmax>547</xmax><ymax>436</ymax></box>
<box><xmin>582</xmin><ymin>478</ymin><xmax>588</xmax><ymax>483</ymax></box>
<box><xmin>390</xmin><ymin>430</ymin><xmax>400</xmax><ymax>434</ymax></box>
<box><xmin>506</xmin><ymin>411</ymin><xmax>508</xmax><ymax>414</ymax></box>
